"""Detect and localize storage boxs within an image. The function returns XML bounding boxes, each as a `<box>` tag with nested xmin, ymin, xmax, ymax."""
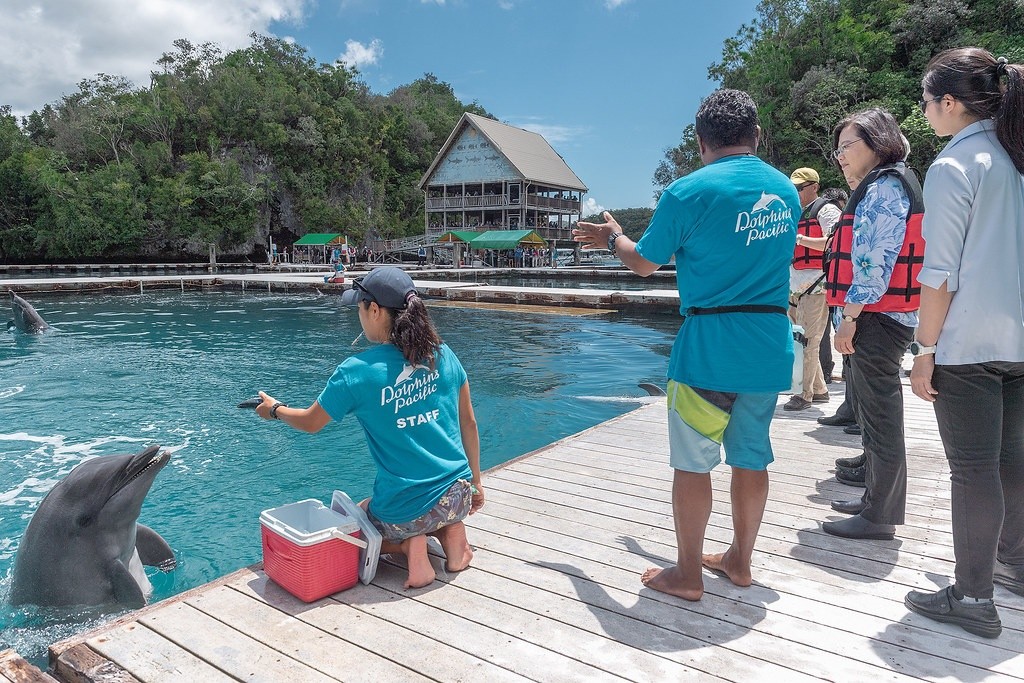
<box><xmin>258</xmin><ymin>490</ymin><xmax>382</xmax><ymax>603</ymax></box>
<box><xmin>334</xmin><ymin>271</ymin><xmax>344</xmax><ymax>283</ymax></box>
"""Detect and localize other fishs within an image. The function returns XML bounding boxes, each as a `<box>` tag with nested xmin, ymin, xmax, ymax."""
<box><xmin>237</xmin><ymin>399</ymin><xmax>263</xmax><ymax>409</ymax></box>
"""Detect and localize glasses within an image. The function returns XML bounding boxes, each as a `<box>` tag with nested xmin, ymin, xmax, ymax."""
<box><xmin>833</xmin><ymin>138</ymin><xmax>863</xmax><ymax>159</ymax></box>
<box><xmin>352</xmin><ymin>277</ymin><xmax>381</xmax><ymax>308</ymax></box>
<box><xmin>797</xmin><ymin>182</ymin><xmax>815</xmax><ymax>192</ymax></box>
<box><xmin>921</xmin><ymin>95</ymin><xmax>945</xmax><ymax>114</ymax></box>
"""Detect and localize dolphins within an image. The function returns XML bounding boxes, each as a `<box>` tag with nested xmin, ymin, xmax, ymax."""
<box><xmin>9</xmin><ymin>443</ymin><xmax>174</xmax><ymax>609</ymax></box>
<box><xmin>7</xmin><ymin>290</ymin><xmax>48</xmax><ymax>330</ymax></box>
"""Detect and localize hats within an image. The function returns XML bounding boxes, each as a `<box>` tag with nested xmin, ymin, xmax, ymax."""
<box><xmin>342</xmin><ymin>267</ymin><xmax>418</xmax><ymax>309</ymax></box>
<box><xmin>790</xmin><ymin>167</ymin><xmax>820</xmax><ymax>185</ymax></box>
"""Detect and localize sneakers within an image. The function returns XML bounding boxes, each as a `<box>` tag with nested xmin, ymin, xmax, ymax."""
<box><xmin>784</xmin><ymin>395</ymin><xmax>812</xmax><ymax>411</ymax></box>
<box><xmin>812</xmin><ymin>391</ymin><xmax>830</xmax><ymax>403</ymax></box>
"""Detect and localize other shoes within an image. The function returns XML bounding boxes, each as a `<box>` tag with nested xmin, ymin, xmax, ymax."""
<box><xmin>835</xmin><ymin>453</ymin><xmax>867</xmax><ymax>468</ymax></box>
<box><xmin>835</xmin><ymin>465</ymin><xmax>867</xmax><ymax>487</ymax></box>
<box><xmin>904</xmin><ymin>369</ymin><xmax>912</xmax><ymax>376</ymax></box>
<box><xmin>824</xmin><ymin>373</ymin><xmax>832</xmax><ymax>385</ymax></box>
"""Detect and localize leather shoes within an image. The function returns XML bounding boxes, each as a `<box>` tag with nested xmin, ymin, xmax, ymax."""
<box><xmin>844</xmin><ymin>424</ymin><xmax>861</xmax><ymax>435</ymax></box>
<box><xmin>822</xmin><ymin>513</ymin><xmax>896</xmax><ymax>540</ymax></box>
<box><xmin>831</xmin><ymin>497</ymin><xmax>866</xmax><ymax>514</ymax></box>
<box><xmin>904</xmin><ymin>584</ymin><xmax>1003</xmax><ymax>638</ymax></box>
<box><xmin>992</xmin><ymin>559</ymin><xmax>1024</xmax><ymax>597</ymax></box>
<box><xmin>818</xmin><ymin>414</ymin><xmax>857</xmax><ymax>426</ymax></box>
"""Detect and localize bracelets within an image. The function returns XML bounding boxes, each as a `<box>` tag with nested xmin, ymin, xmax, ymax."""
<box><xmin>270</xmin><ymin>402</ymin><xmax>288</xmax><ymax>420</ymax></box>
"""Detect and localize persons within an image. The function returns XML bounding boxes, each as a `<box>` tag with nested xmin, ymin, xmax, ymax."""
<box><xmin>255</xmin><ymin>266</ymin><xmax>485</xmax><ymax>590</ymax></box>
<box><xmin>817</xmin><ymin>134</ymin><xmax>911</xmax><ymax>435</ymax></box>
<box><xmin>904</xmin><ymin>46</ymin><xmax>1024</xmax><ymax>638</ymax></box>
<box><xmin>358</xmin><ymin>246</ymin><xmax>372</xmax><ymax>263</ymax></box>
<box><xmin>823</xmin><ymin>104</ymin><xmax>924</xmax><ymax>541</ymax></box>
<box><xmin>264</xmin><ymin>244</ymin><xmax>269</xmax><ymax>263</ymax></box>
<box><xmin>430</xmin><ymin>219</ymin><xmax>567</xmax><ymax>230</ymax></box>
<box><xmin>418</xmin><ymin>246</ymin><xmax>426</xmax><ymax>267</ymax></box>
<box><xmin>431</xmin><ymin>190</ymin><xmax>578</xmax><ymax>201</ymax></box>
<box><xmin>327</xmin><ymin>260</ymin><xmax>345</xmax><ymax>282</ymax></box>
<box><xmin>819</xmin><ymin>188</ymin><xmax>849</xmax><ymax>383</ymax></box>
<box><xmin>784</xmin><ymin>166</ymin><xmax>843</xmax><ymax>411</ymax></box>
<box><xmin>572</xmin><ymin>87</ymin><xmax>803</xmax><ymax>601</ymax></box>
<box><xmin>272</xmin><ymin>245</ymin><xmax>356</xmax><ymax>267</ymax></box>
<box><xmin>482</xmin><ymin>245</ymin><xmax>546</xmax><ymax>267</ymax></box>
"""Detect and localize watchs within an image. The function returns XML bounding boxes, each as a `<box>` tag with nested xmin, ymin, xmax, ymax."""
<box><xmin>842</xmin><ymin>314</ymin><xmax>857</xmax><ymax>322</ymax></box>
<box><xmin>911</xmin><ymin>341</ymin><xmax>936</xmax><ymax>358</ymax></box>
<box><xmin>607</xmin><ymin>232</ymin><xmax>625</xmax><ymax>253</ymax></box>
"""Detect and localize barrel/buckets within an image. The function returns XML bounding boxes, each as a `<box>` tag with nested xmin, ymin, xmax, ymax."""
<box><xmin>334</xmin><ymin>277</ymin><xmax>345</xmax><ymax>282</ymax></box>
<box><xmin>258</xmin><ymin>498</ymin><xmax>366</xmax><ymax>603</ymax></box>
